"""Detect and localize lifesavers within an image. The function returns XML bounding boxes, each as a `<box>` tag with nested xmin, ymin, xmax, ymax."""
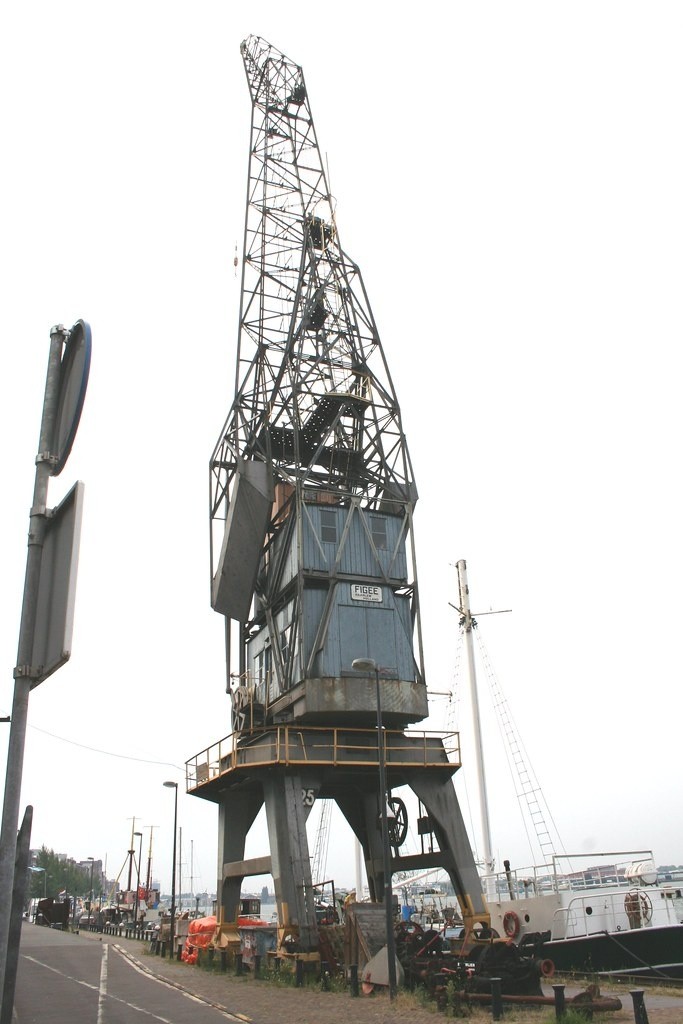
<box><xmin>503</xmin><ymin>911</ymin><xmax>521</xmax><ymax>938</ymax></box>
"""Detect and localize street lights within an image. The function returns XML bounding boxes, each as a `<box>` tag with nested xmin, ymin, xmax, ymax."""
<box><xmin>161</xmin><ymin>780</ymin><xmax>179</xmax><ymax>961</ymax></box>
<box><xmin>134</xmin><ymin>831</ymin><xmax>142</xmax><ymax>940</ymax></box>
<box><xmin>88</xmin><ymin>856</ymin><xmax>93</xmax><ymax>932</ymax></box>
<box><xmin>63</xmin><ymin>867</ymin><xmax>68</xmax><ymax>930</ymax></box>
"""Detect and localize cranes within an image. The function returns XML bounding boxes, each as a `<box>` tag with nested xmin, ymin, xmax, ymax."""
<box><xmin>181</xmin><ymin>34</ymin><xmax>495</xmax><ymax>973</ymax></box>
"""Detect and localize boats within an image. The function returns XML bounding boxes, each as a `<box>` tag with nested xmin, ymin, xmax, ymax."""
<box><xmin>392</xmin><ymin>548</ymin><xmax>683</xmax><ymax>985</ymax></box>
